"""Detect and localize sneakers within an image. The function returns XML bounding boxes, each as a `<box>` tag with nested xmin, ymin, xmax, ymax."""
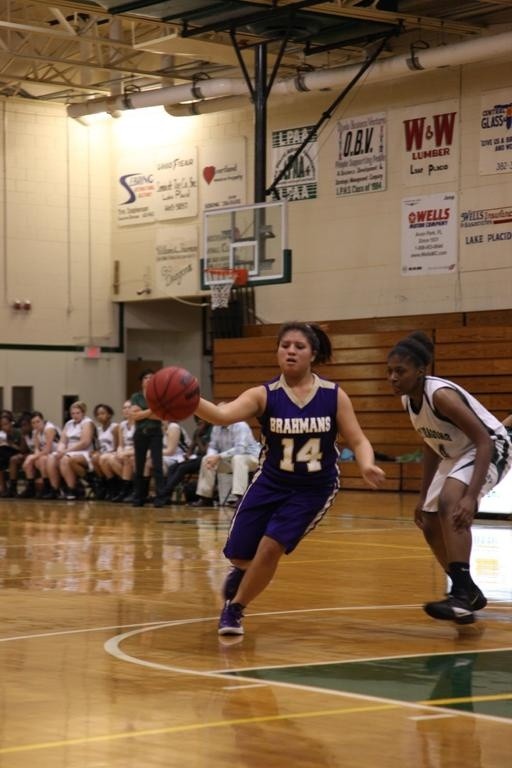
<box><xmin>218</xmin><ymin>598</ymin><xmax>246</xmax><ymax>635</ymax></box>
<box><xmin>424</xmin><ymin>583</ymin><xmax>487</xmax><ymax>625</ymax></box>
<box><xmin>221</xmin><ymin>566</ymin><xmax>247</xmax><ymax>599</ymax></box>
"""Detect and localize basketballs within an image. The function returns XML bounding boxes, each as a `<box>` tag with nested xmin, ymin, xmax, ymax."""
<box><xmin>146</xmin><ymin>366</ymin><xmax>200</xmax><ymax>419</ymax></box>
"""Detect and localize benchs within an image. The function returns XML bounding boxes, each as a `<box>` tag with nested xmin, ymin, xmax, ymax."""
<box><xmin>338</xmin><ymin>459</ymin><xmax>425</xmax><ymax>491</ymax></box>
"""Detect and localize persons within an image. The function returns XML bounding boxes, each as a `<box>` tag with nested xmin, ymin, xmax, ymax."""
<box><xmin>386</xmin><ymin>330</ymin><xmax>512</xmax><ymax>625</ymax></box>
<box><xmin>193</xmin><ymin>322</ymin><xmax>386</xmax><ymax>637</ymax></box>
<box><xmin>0</xmin><ymin>368</ymin><xmax>265</xmax><ymax>509</ymax></box>
<box><xmin>0</xmin><ymin>499</ymin><xmax>236</xmax><ymax>609</ymax></box>
<box><xmin>218</xmin><ymin>632</ymin><xmax>330</xmax><ymax>768</ymax></box>
<box><xmin>415</xmin><ymin>620</ymin><xmax>487</xmax><ymax>767</ymax></box>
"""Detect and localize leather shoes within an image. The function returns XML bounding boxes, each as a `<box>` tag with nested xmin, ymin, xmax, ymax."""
<box><xmin>192</xmin><ymin>498</ymin><xmax>214</xmax><ymax>507</ymax></box>
